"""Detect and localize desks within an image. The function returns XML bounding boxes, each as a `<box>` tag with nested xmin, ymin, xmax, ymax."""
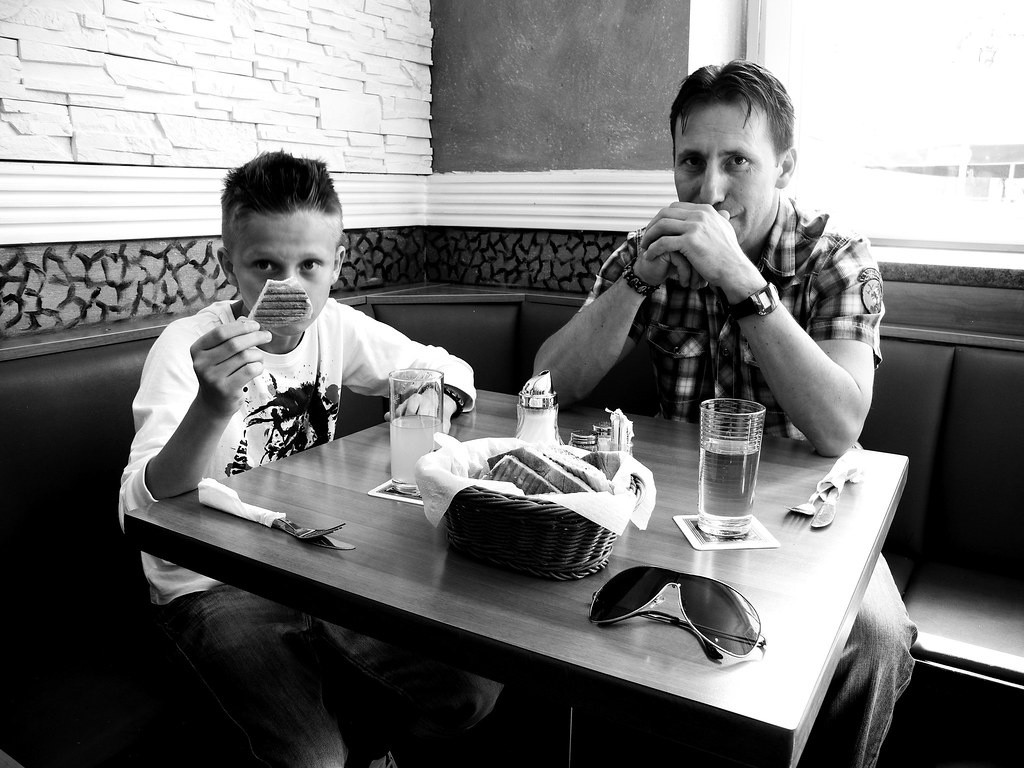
<box><xmin>125</xmin><ymin>385</ymin><xmax>909</xmax><ymax>768</ymax></box>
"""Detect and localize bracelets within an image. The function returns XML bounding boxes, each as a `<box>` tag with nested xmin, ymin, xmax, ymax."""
<box><xmin>622</xmin><ymin>257</ymin><xmax>661</xmax><ymax>297</ymax></box>
<box><xmin>418</xmin><ymin>383</ymin><xmax>465</xmax><ymax>419</ymax></box>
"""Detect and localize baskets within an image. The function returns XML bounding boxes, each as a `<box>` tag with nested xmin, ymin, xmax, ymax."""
<box><xmin>425</xmin><ymin>437</ymin><xmax>641</xmax><ymax>581</ymax></box>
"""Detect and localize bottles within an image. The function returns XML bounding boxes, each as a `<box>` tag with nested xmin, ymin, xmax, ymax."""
<box><xmin>513</xmin><ymin>369</ymin><xmax>566</xmax><ymax>447</ymax></box>
<box><xmin>568</xmin><ymin>421</ymin><xmax>613</xmax><ymax>455</ymax></box>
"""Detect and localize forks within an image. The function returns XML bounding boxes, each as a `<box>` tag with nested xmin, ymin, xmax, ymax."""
<box><xmin>786</xmin><ymin>483</ymin><xmax>833</xmax><ymax>517</ymax></box>
<box><xmin>279</xmin><ymin>517</ymin><xmax>346</xmax><ymax>541</ymax></box>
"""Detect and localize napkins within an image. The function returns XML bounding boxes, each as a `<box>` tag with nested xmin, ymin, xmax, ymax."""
<box><xmin>817</xmin><ymin>448</ymin><xmax>864</xmax><ymax>500</ymax></box>
<box><xmin>196</xmin><ymin>477</ymin><xmax>286</xmax><ymax>529</ymax></box>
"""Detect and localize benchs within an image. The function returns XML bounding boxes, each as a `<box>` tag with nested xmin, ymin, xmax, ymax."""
<box><xmin>0</xmin><ymin>284</ymin><xmax>1024</xmax><ymax>767</ymax></box>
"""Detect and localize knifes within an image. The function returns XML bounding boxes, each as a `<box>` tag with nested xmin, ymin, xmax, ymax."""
<box><xmin>273</xmin><ymin>519</ymin><xmax>355</xmax><ymax>552</ymax></box>
<box><xmin>809</xmin><ymin>486</ymin><xmax>838</xmax><ymax>528</ymax></box>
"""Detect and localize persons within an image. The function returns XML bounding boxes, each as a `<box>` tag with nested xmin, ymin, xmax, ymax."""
<box><xmin>532</xmin><ymin>59</ymin><xmax>918</xmax><ymax>768</ymax></box>
<box><xmin>118</xmin><ymin>147</ymin><xmax>506</xmax><ymax>768</ymax></box>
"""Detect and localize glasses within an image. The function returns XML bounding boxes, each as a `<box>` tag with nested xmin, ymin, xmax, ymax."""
<box><xmin>585</xmin><ymin>565</ymin><xmax>770</xmax><ymax>664</ymax></box>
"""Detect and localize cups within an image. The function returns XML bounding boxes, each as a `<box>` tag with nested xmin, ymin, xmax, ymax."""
<box><xmin>697</xmin><ymin>398</ymin><xmax>766</xmax><ymax>538</ymax></box>
<box><xmin>388</xmin><ymin>368</ymin><xmax>445</xmax><ymax>498</ymax></box>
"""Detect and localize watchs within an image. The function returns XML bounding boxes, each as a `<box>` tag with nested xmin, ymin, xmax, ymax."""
<box><xmin>728</xmin><ymin>282</ymin><xmax>779</xmax><ymax>320</ymax></box>
